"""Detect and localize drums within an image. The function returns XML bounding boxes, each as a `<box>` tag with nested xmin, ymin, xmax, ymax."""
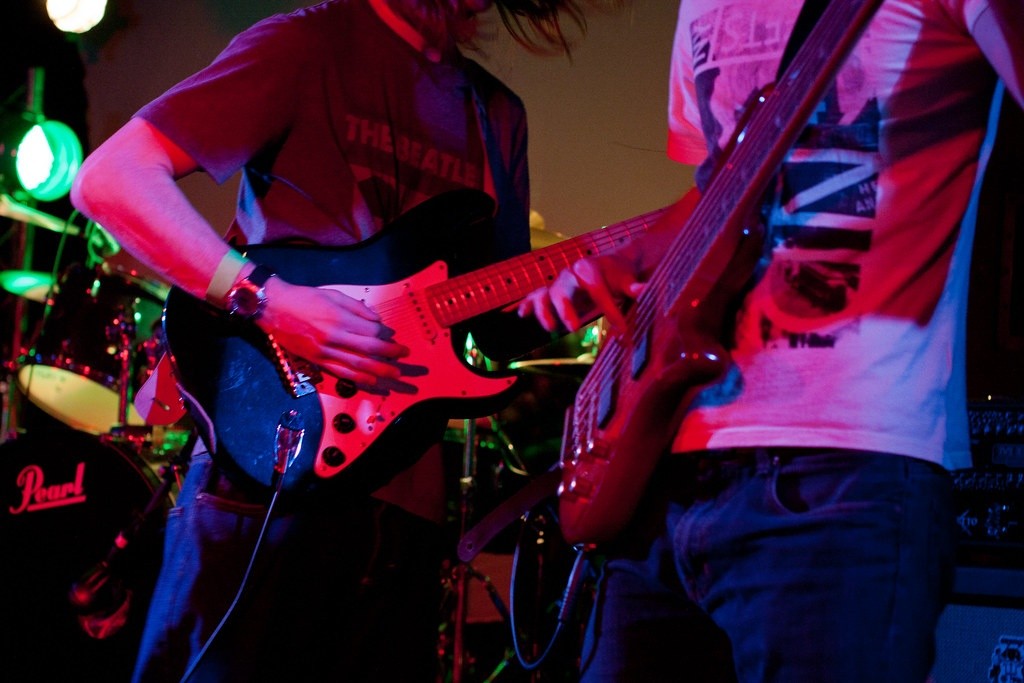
<box><xmin>14</xmin><ymin>262</ymin><xmax>178</xmax><ymax>452</ymax></box>
<box><xmin>0</xmin><ymin>423</ymin><xmax>204</xmax><ymax>683</ymax></box>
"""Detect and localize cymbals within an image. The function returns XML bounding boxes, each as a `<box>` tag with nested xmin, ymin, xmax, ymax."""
<box><xmin>508</xmin><ymin>352</ymin><xmax>603</xmax><ymax>376</ymax></box>
<box><xmin>0</xmin><ymin>194</ymin><xmax>79</xmax><ymax>238</ymax></box>
<box><xmin>0</xmin><ymin>268</ymin><xmax>61</xmax><ymax>304</ymax></box>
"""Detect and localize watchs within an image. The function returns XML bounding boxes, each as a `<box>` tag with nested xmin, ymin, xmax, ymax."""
<box><xmin>224</xmin><ymin>264</ymin><xmax>276</xmax><ymax>321</ymax></box>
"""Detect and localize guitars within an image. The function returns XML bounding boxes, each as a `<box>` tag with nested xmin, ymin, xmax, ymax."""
<box><xmin>559</xmin><ymin>0</ymin><xmax>885</xmax><ymax>544</ymax></box>
<box><xmin>159</xmin><ymin>197</ymin><xmax>701</xmax><ymax>511</ymax></box>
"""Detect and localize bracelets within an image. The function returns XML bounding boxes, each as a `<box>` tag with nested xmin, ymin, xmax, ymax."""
<box><xmin>207</xmin><ymin>248</ymin><xmax>250</xmax><ymax>308</ymax></box>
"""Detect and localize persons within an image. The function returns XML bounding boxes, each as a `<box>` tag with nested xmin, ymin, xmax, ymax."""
<box><xmin>517</xmin><ymin>0</ymin><xmax>1024</xmax><ymax>683</ymax></box>
<box><xmin>70</xmin><ymin>0</ymin><xmax>633</xmax><ymax>683</ymax></box>
